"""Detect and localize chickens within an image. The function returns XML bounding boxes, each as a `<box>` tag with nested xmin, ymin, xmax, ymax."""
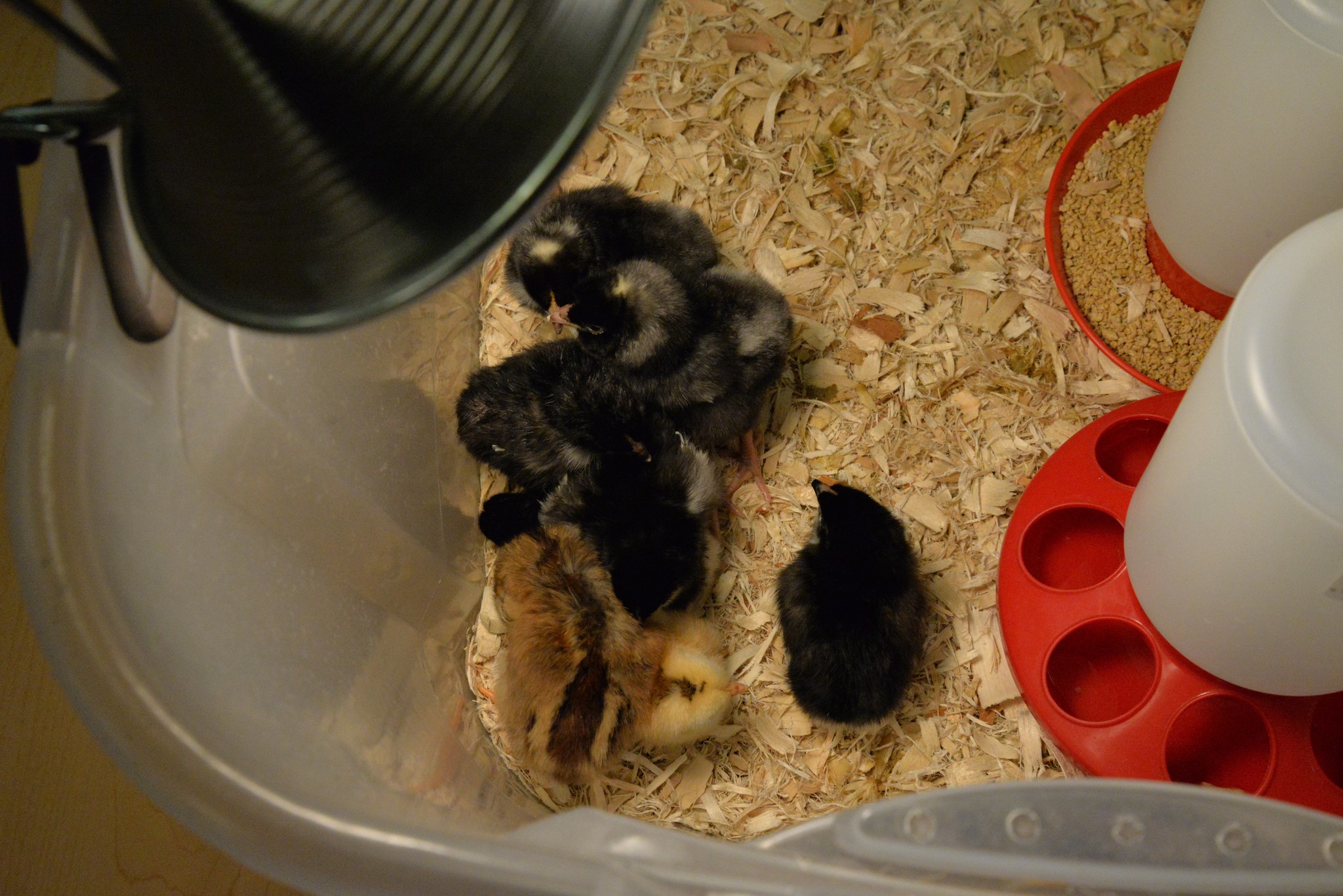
<box><xmin>447</xmin><ymin>177</ymin><xmax>800</xmax><ymax>791</ymax></box>
<box><xmin>770</xmin><ymin>470</ymin><xmax>932</xmax><ymax>729</ymax></box>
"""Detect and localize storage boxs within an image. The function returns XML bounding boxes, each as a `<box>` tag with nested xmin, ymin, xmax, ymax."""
<box><xmin>5</xmin><ymin>0</ymin><xmax>1343</xmax><ymax>896</ymax></box>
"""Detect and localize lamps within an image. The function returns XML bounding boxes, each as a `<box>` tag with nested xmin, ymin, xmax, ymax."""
<box><xmin>0</xmin><ymin>0</ymin><xmax>656</xmax><ymax>343</ymax></box>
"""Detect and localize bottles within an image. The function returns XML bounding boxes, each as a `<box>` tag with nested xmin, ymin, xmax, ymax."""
<box><xmin>1121</xmin><ymin>209</ymin><xmax>1343</xmax><ymax>698</ymax></box>
<box><xmin>1137</xmin><ymin>0</ymin><xmax>1343</xmax><ymax>297</ymax></box>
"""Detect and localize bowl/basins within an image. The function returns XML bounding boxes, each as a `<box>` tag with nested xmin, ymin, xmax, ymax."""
<box><xmin>1044</xmin><ymin>58</ymin><xmax>1224</xmax><ymax>393</ymax></box>
<box><xmin>996</xmin><ymin>392</ymin><xmax>1343</xmax><ymax>821</ymax></box>
